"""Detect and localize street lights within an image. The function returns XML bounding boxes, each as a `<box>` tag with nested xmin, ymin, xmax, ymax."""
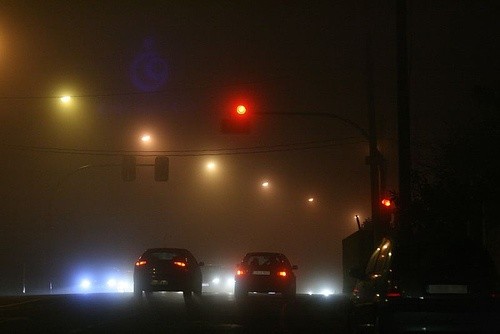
<box><xmin>233</xmin><ymin>97</ymin><xmax>378</xmax><ymax>163</ymax></box>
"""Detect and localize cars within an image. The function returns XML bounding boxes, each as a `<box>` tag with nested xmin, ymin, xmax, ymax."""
<box><xmin>349</xmin><ymin>228</ymin><xmax>499</xmax><ymax>333</ymax></box>
<box><xmin>133</xmin><ymin>248</ymin><xmax>205</xmax><ymax>300</ymax></box>
<box><xmin>235</xmin><ymin>252</ymin><xmax>298</xmax><ymax>298</ymax></box>
